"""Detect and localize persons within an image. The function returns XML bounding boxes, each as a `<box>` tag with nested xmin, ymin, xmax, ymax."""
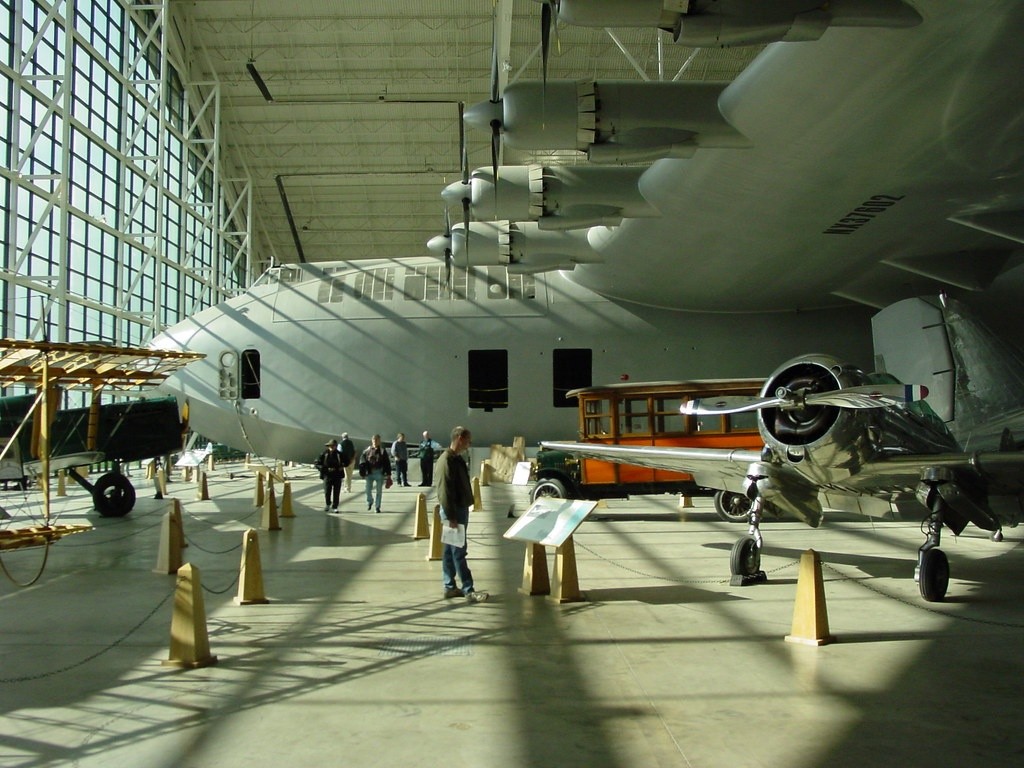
<box><xmin>120</xmin><ymin>462</ymin><xmax>133</xmax><ymax>477</ymax></box>
<box><xmin>391</xmin><ymin>433</ymin><xmax>411</xmax><ymax>487</ymax></box>
<box><xmin>414</xmin><ymin>431</ymin><xmax>442</xmax><ymax>486</ymax></box>
<box><xmin>435</xmin><ymin>426</ymin><xmax>489</xmax><ymax>601</ymax></box>
<box><xmin>337</xmin><ymin>432</ymin><xmax>355</xmax><ymax>492</ymax></box>
<box><xmin>315</xmin><ymin>439</ymin><xmax>349</xmax><ymax>512</ymax></box>
<box><xmin>360</xmin><ymin>434</ymin><xmax>391</xmax><ymax>512</ymax></box>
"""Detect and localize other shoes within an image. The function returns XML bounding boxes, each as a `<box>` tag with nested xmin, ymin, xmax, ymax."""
<box><xmin>465</xmin><ymin>592</ymin><xmax>488</xmax><ymax>604</ymax></box>
<box><xmin>403</xmin><ymin>482</ymin><xmax>411</xmax><ymax>486</ymax></box>
<box><xmin>444</xmin><ymin>589</ymin><xmax>464</xmax><ymax>599</ymax></box>
<box><xmin>325</xmin><ymin>503</ymin><xmax>330</xmax><ymax>511</ymax></box>
<box><xmin>419</xmin><ymin>482</ymin><xmax>431</xmax><ymax>487</ymax></box>
<box><xmin>367</xmin><ymin>503</ymin><xmax>372</xmax><ymax>511</ymax></box>
<box><xmin>376</xmin><ymin>507</ymin><xmax>380</xmax><ymax>513</ymax></box>
<box><xmin>333</xmin><ymin>508</ymin><xmax>338</xmax><ymax>513</ymax></box>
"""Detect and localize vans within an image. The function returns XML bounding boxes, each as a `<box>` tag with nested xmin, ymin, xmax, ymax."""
<box><xmin>528</xmin><ymin>378</ymin><xmax>769</xmax><ymax>524</ymax></box>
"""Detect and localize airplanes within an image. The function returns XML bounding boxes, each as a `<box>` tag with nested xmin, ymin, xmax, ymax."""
<box><xmin>62</xmin><ymin>0</ymin><xmax>1023</xmax><ymax>525</ymax></box>
<box><xmin>514</xmin><ymin>353</ymin><xmax>1024</xmax><ymax>597</ymax></box>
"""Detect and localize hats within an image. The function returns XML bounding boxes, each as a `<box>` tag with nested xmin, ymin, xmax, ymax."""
<box><xmin>325</xmin><ymin>439</ymin><xmax>337</xmax><ymax>446</ymax></box>
